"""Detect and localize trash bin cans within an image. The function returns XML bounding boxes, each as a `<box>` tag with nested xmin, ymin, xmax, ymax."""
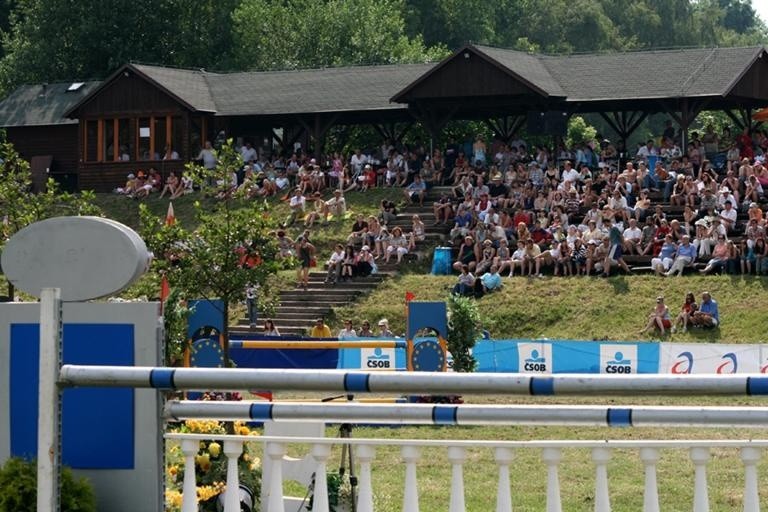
<box><xmin>432</xmin><ymin>247</ymin><xmax>453</xmax><ymax>274</ymax></box>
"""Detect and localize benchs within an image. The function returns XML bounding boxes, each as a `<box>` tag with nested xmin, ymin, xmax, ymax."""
<box><xmin>473</xmin><ymin>173</ymin><xmax>768</xmax><ymax>265</ymax></box>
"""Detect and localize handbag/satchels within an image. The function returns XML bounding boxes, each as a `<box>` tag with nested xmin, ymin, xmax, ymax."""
<box><xmin>309</xmin><ymin>259</ymin><xmax>317</xmax><ymax>267</ymax></box>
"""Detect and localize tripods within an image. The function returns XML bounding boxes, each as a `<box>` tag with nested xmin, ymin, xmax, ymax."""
<box><xmin>297</xmin><ymin>424</ymin><xmax>358</xmax><ymax>512</ymax></box>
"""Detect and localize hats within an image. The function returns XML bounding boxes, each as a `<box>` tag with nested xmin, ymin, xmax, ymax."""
<box><xmin>694</xmin><ymin>219</ymin><xmax>708</xmax><ymax>229</ymax></box>
<box><xmin>528</xmin><ymin>161</ymin><xmax>538</xmax><ymax>166</ymax></box>
<box><xmin>333</xmin><ymin>189</ymin><xmax>342</xmax><ymax>195</ymax></box>
<box><xmin>656</xmin><ymin>297</ymin><xmax>663</xmax><ymax>303</ymax></box>
<box><xmin>492</xmin><ymin>175</ymin><xmax>501</xmax><ymax>181</ymax></box>
<box><xmin>362</xmin><ymin>245</ymin><xmax>370</xmax><ymax>250</ymax></box>
<box><xmin>587</xmin><ymin>239</ymin><xmax>599</xmax><ymax>247</ymax></box>
<box><xmin>721</xmin><ymin>187</ymin><xmax>732</xmax><ymax>194</ymax></box>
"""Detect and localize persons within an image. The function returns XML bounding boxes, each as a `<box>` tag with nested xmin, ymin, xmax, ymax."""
<box><xmin>120</xmin><ymin>118</ymin><xmax>767</xmax><ymax>337</ymax></box>
